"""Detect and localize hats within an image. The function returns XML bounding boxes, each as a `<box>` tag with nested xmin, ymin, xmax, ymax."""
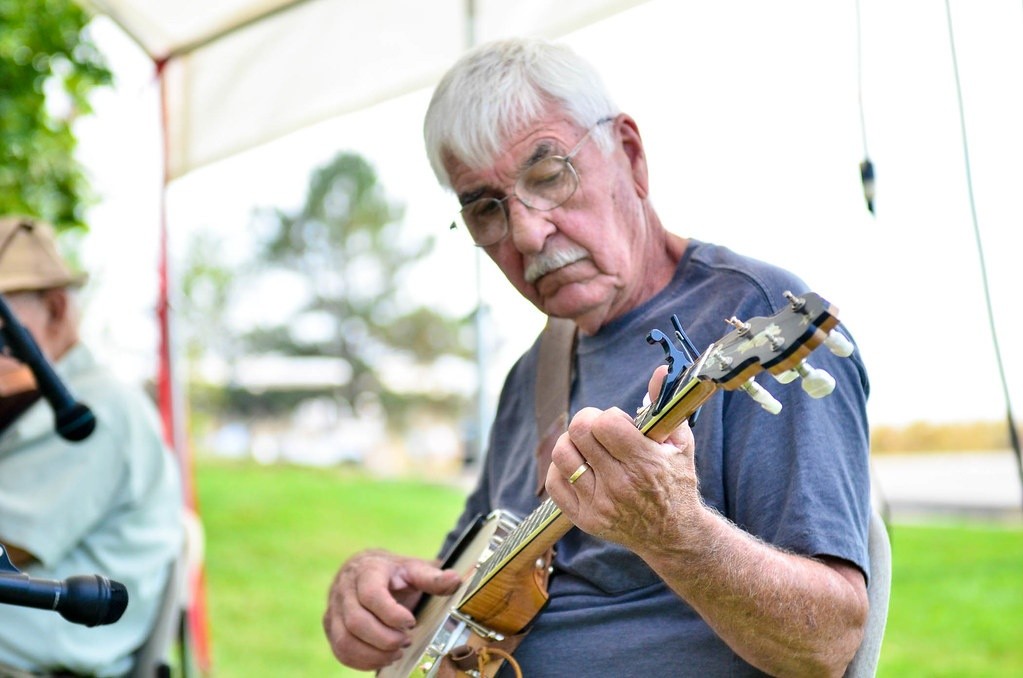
<box><xmin>0</xmin><ymin>209</ymin><xmax>86</xmax><ymax>294</ymax></box>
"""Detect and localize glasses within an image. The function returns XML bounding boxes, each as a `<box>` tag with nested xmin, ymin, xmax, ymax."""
<box><xmin>447</xmin><ymin>113</ymin><xmax>613</xmax><ymax>248</ymax></box>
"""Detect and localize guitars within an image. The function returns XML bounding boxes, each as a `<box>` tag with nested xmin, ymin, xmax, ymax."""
<box><xmin>371</xmin><ymin>291</ymin><xmax>854</xmax><ymax>678</ymax></box>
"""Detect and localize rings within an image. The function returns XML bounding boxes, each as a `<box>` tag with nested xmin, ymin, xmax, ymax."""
<box><xmin>568</xmin><ymin>462</ymin><xmax>590</xmax><ymax>484</ymax></box>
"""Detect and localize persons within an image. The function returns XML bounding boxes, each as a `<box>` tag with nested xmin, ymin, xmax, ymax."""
<box><xmin>0</xmin><ymin>212</ymin><xmax>194</xmax><ymax>677</ymax></box>
<box><xmin>322</xmin><ymin>41</ymin><xmax>871</xmax><ymax>678</ymax></box>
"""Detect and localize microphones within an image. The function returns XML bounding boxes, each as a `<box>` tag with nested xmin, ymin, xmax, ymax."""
<box><xmin>1</xmin><ymin>297</ymin><xmax>97</xmax><ymax>442</ymax></box>
<box><xmin>0</xmin><ymin>571</ymin><xmax>128</xmax><ymax>629</ymax></box>
